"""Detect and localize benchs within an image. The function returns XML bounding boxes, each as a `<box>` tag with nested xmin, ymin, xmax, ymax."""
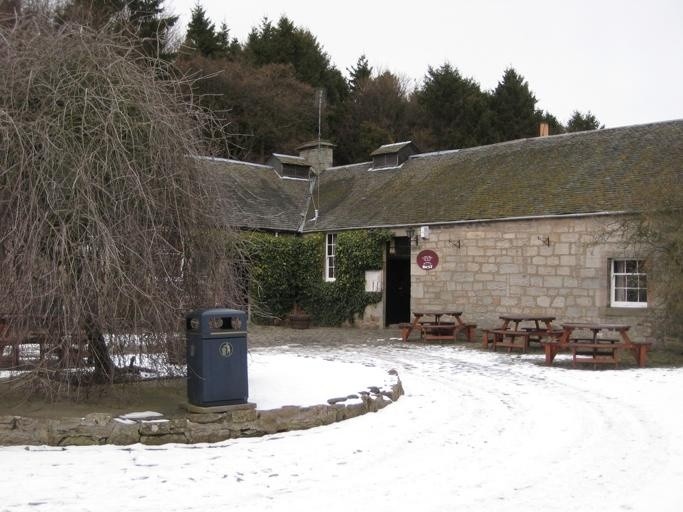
<box><xmin>397</xmin><ymin>307</ymin><xmax>650</xmax><ymax>369</ymax></box>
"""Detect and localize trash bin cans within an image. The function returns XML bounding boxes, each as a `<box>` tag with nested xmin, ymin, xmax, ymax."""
<box><xmin>185</xmin><ymin>308</ymin><xmax>249</xmax><ymax>407</ymax></box>
